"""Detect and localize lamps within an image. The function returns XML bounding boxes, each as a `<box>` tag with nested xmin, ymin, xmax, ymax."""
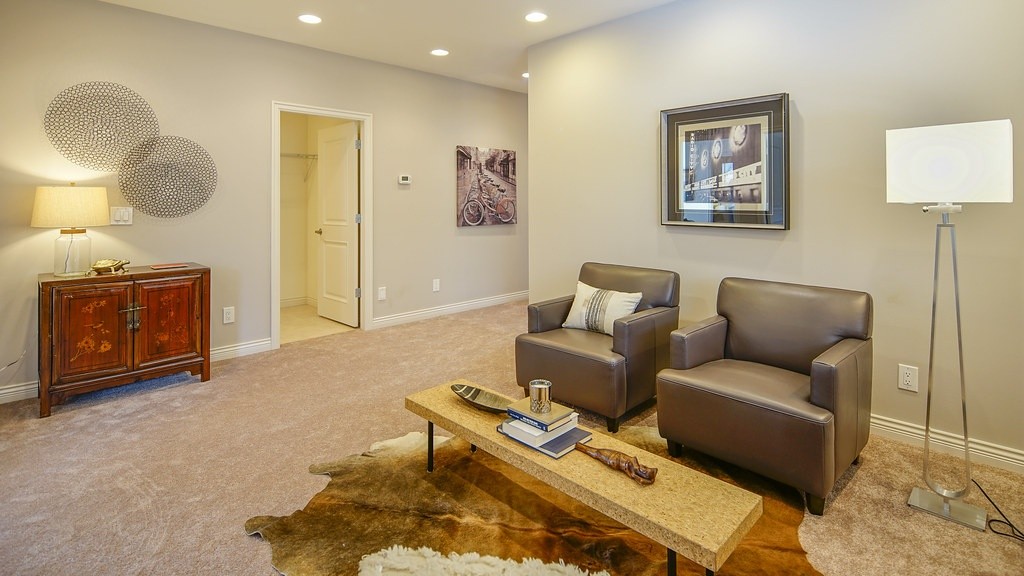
<box><xmin>30</xmin><ymin>183</ymin><xmax>110</xmax><ymax>277</ymax></box>
<box><xmin>886</xmin><ymin>119</ymin><xmax>1024</xmax><ymax>543</ymax></box>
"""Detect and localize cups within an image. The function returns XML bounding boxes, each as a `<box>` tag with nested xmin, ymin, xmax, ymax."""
<box><xmin>528</xmin><ymin>379</ymin><xmax>553</xmax><ymax>414</ymax></box>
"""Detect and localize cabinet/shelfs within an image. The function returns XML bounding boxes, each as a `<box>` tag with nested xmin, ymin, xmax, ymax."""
<box><xmin>37</xmin><ymin>262</ymin><xmax>212</xmax><ymax>419</ymax></box>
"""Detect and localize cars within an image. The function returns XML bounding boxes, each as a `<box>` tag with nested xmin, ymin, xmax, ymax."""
<box><xmin>703</xmin><ymin>195</ymin><xmax>719</xmax><ymax>203</ymax></box>
<box><xmin>685</xmin><ymin>192</ymin><xmax>695</xmax><ymax>201</ymax></box>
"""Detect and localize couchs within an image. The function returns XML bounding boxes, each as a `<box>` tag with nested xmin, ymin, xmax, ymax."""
<box><xmin>513</xmin><ymin>261</ymin><xmax>682</xmax><ymax>432</ymax></box>
<box><xmin>655</xmin><ymin>276</ymin><xmax>873</xmax><ymax>519</ymax></box>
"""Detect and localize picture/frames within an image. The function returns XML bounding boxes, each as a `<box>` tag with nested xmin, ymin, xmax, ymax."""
<box><xmin>660</xmin><ymin>91</ymin><xmax>792</xmax><ymax>230</ymax></box>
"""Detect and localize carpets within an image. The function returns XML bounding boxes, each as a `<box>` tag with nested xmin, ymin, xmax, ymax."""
<box><xmin>247</xmin><ymin>429</ymin><xmax>826</xmax><ymax>576</ymax></box>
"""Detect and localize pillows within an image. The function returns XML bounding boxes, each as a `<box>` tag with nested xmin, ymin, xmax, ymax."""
<box><xmin>561</xmin><ymin>280</ymin><xmax>642</xmax><ymax>336</ymax></box>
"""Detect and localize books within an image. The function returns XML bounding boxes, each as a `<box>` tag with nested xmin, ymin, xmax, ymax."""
<box><xmin>497</xmin><ymin>396</ymin><xmax>592</xmax><ymax>459</ymax></box>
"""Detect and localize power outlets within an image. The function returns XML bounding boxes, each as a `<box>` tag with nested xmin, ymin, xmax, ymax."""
<box><xmin>898</xmin><ymin>364</ymin><xmax>918</xmax><ymax>393</ymax></box>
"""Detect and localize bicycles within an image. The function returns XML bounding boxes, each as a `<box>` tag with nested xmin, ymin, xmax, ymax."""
<box><xmin>462</xmin><ymin>170</ymin><xmax>515</xmax><ymax>226</ymax></box>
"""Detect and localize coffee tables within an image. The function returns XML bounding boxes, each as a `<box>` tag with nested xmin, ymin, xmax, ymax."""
<box><xmin>404</xmin><ymin>378</ymin><xmax>764</xmax><ymax>576</ymax></box>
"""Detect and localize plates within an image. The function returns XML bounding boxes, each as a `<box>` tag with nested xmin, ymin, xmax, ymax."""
<box><xmin>450</xmin><ymin>383</ymin><xmax>515</xmax><ymax>414</ymax></box>
